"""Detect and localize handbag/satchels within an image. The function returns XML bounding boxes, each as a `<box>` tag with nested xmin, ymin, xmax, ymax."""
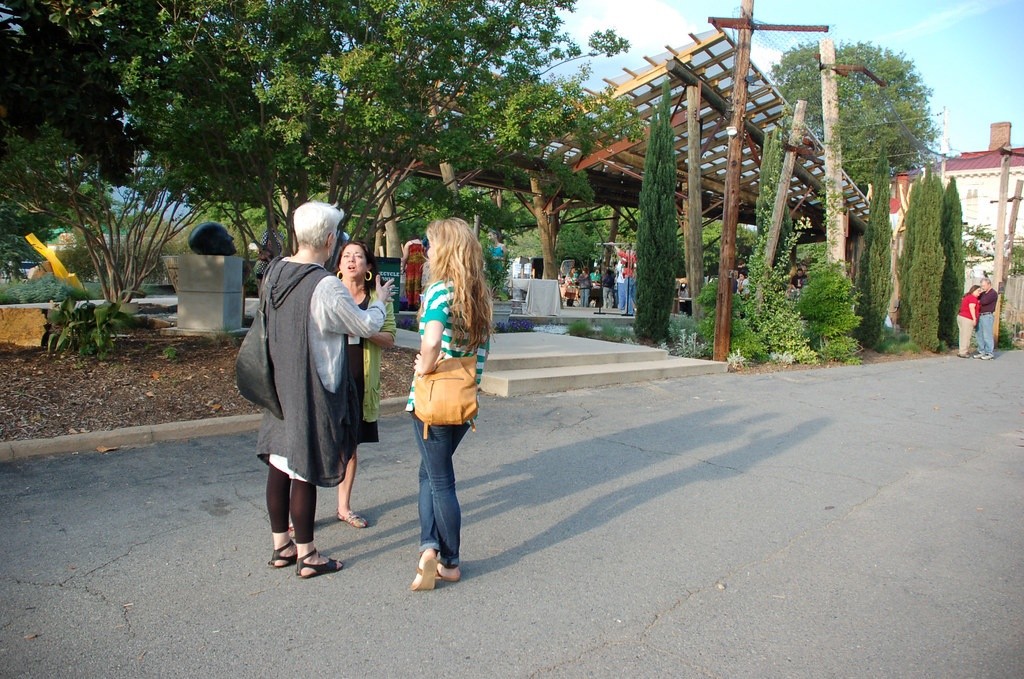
<box><xmin>234</xmin><ymin>307</ymin><xmax>285</xmax><ymax>420</ymax></box>
<box><xmin>414</xmin><ymin>354</ymin><xmax>480</xmax><ymax>440</ymax></box>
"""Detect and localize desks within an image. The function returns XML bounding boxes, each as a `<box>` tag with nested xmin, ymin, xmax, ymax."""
<box><xmin>511</xmin><ymin>278</ymin><xmax>562</xmax><ymax>316</ymax></box>
<box><xmin>673</xmin><ymin>298</ymin><xmax>692</xmax><ymax>317</ymax></box>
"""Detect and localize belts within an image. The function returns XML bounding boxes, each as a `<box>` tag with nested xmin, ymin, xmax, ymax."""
<box><xmin>980</xmin><ymin>313</ymin><xmax>990</xmax><ymax>315</ymax></box>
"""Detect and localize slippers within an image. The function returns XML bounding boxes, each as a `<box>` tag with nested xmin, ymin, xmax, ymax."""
<box><xmin>434</xmin><ymin>559</ymin><xmax>460</xmax><ymax>581</ymax></box>
<box><xmin>409</xmin><ymin>558</ymin><xmax>437</xmax><ymax>590</ymax></box>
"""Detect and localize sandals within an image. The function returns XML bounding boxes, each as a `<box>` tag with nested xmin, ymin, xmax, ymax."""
<box><xmin>295</xmin><ymin>547</ymin><xmax>343</xmax><ymax>578</ymax></box>
<box><xmin>268</xmin><ymin>539</ymin><xmax>298</xmax><ymax>567</ymax></box>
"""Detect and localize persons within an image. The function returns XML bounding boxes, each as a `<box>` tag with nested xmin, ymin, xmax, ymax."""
<box><xmin>402</xmin><ymin>234</ymin><xmax>430</xmax><ymax>310</ymax></box>
<box><xmin>564</xmin><ymin>241</ymin><xmax>808</xmax><ymax>316</ymax></box>
<box><xmin>957</xmin><ymin>277</ymin><xmax>998</xmax><ymax>360</ymax></box>
<box><xmin>255</xmin><ymin>199</ymin><xmax>398</xmax><ymax>579</ymax></box>
<box><xmin>405</xmin><ymin>216</ymin><xmax>493</xmax><ymax>592</ymax></box>
<box><xmin>486</xmin><ymin>227</ymin><xmax>509</xmax><ymax>269</ymax></box>
<box><xmin>187</xmin><ymin>221</ymin><xmax>237</xmax><ymax>255</ymax></box>
<box><xmin>287</xmin><ymin>239</ymin><xmax>396</xmax><ymax>538</ymax></box>
<box><xmin>254</xmin><ymin>213</ymin><xmax>287</xmax><ymax>295</ymax></box>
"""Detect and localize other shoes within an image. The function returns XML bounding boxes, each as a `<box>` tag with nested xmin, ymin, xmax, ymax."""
<box><xmin>288</xmin><ymin>526</ymin><xmax>296</xmax><ymax>537</ymax></box>
<box><xmin>980</xmin><ymin>352</ymin><xmax>994</xmax><ymax>360</ymax></box>
<box><xmin>957</xmin><ymin>353</ymin><xmax>970</xmax><ymax>358</ymax></box>
<box><xmin>336</xmin><ymin>507</ymin><xmax>368</xmax><ymax>528</ymax></box>
<box><xmin>973</xmin><ymin>350</ymin><xmax>985</xmax><ymax>358</ymax></box>
